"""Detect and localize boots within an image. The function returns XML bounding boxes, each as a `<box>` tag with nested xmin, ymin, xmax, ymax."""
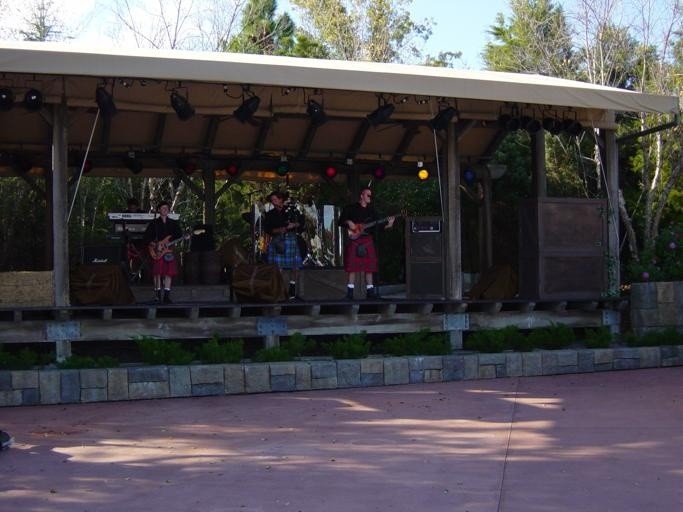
<box><xmin>153</xmin><ymin>289</ymin><xmax>161</xmax><ymax>302</ymax></box>
<box><xmin>366</xmin><ymin>287</ymin><xmax>382</xmax><ymax>301</ymax></box>
<box><xmin>343</xmin><ymin>287</ymin><xmax>354</xmax><ymax>301</ymax></box>
<box><xmin>288</xmin><ymin>283</ymin><xmax>297</xmax><ymax>303</ymax></box>
<box><xmin>164</xmin><ymin>289</ymin><xmax>173</xmax><ymax>304</ymax></box>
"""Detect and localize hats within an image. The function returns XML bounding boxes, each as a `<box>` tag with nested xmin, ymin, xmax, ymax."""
<box><xmin>156</xmin><ymin>201</ymin><xmax>171</xmax><ymax>214</ymax></box>
<box><xmin>266</xmin><ymin>191</ymin><xmax>289</xmax><ymax>203</ymax></box>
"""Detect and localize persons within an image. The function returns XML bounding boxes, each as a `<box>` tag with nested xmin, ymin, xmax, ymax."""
<box><xmin>120</xmin><ymin>200</ymin><xmax>152</xmax><ymax>274</ymax></box>
<box><xmin>335</xmin><ymin>185</ymin><xmax>397</xmax><ymax>301</ymax></box>
<box><xmin>141</xmin><ymin>200</ymin><xmax>192</xmax><ymax>305</ymax></box>
<box><xmin>260</xmin><ymin>192</ymin><xmax>304</xmax><ymax>303</ymax></box>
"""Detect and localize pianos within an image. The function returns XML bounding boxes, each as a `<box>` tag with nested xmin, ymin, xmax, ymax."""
<box><xmin>108</xmin><ymin>212</ymin><xmax>180</xmax><ymax>234</ymax></box>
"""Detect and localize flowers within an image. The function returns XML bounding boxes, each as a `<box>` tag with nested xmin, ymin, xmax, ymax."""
<box><xmin>628</xmin><ymin>220</ymin><xmax>682</xmax><ymax>282</ymax></box>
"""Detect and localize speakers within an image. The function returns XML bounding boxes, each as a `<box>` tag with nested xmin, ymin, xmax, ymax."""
<box><xmin>518</xmin><ymin>197</ymin><xmax>608</xmax><ymax>299</ymax></box>
<box><xmin>230</xmin><ymin>263</ymin><xmax>289</xmax><ymax>302</ymax></box>
<box><xmin>70</xmin><ymin>261</ymin><xmax>135</xmax><ymax>304</ymax></box>
<box><xmin>404</xmin><ymin>216</ymin><xmax>445</xmax><ymax>299</ymax></box>
<box><xmin>296</xmin><ymin>268</ymin><xmax>348</xmax><ymax>300</ymax></box>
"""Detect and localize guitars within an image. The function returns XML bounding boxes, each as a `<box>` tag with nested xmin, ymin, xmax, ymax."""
<box><xmin>348</xmin><ymin>215</ymin><xmax>401</xmax><ymax>240</ymax></box>
<box><xmin>149</xmin><ymin>230</ymin><xmax>205</xmax><ymax>260</ymax></box>
